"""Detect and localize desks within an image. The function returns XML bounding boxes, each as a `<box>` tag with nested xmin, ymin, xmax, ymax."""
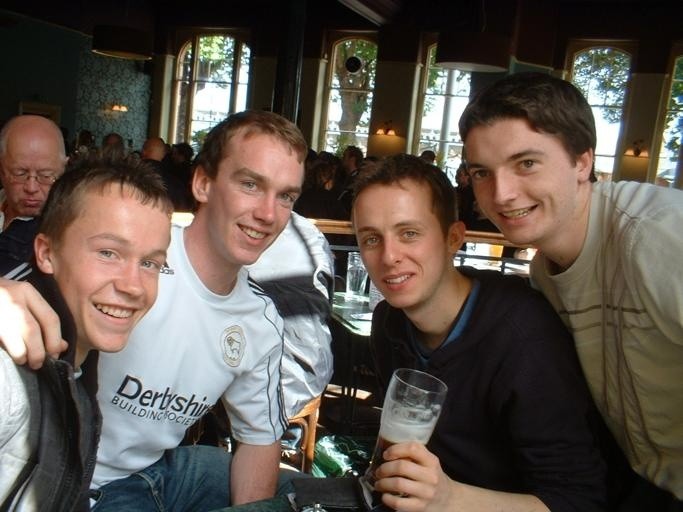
<box><xmin>322</xmin><ymin>292</ymin><xmax>379</xmax><ymax>438</ymax></box>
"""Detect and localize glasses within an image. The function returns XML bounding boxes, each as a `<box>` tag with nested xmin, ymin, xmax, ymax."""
<box><xmin>0</xmin><ymin>171</ymin><xmax>61</xmax><ymax>185</ymax></box>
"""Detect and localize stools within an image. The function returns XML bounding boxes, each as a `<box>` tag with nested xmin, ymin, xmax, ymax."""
<box><xmin>220</xmin><ymin>397</ymin><xmax>322</xmax><ymax>480</ymax></box>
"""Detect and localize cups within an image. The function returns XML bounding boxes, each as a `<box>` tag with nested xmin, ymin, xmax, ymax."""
<box><xmin>343</xmin><ymin>251</ymin><xmax>368</xmax><ymax>300</ymax></box>
<box><xmin>360</xmin><ymin>367</ymin><xmax>448</xmax><ymax>498</ymax></box>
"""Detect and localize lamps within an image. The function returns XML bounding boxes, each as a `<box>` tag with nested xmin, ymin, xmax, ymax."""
<box><xmin>432</xmin><ymin>1</ymin><xmax>509</xmax><ymax>75</ymax></box>
<box><xmin>375</xmin><ymin>119</ymin><xmax>396</xmax><ymax>137</ymax></box>
<box><xmin>90</xmin><ymin>1</ymin><xmax>156</xmax><ymax>61</ymax></box>
<box><xmin>624</xmin><ymin>139</ymin><xmax>650</xmax><ymax>159</ymax></box>
